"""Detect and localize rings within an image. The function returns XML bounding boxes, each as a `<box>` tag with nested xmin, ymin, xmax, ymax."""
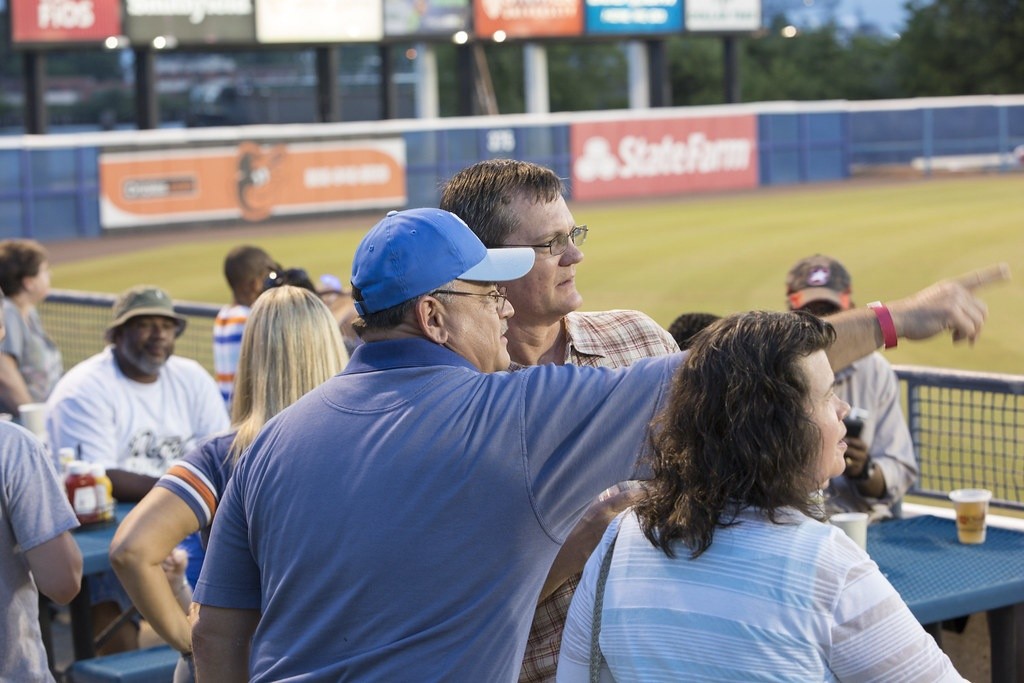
<box><xmin>846</xmin><ymin>457</ymin><xmax>852</xmax><ymax>466</ymax></box>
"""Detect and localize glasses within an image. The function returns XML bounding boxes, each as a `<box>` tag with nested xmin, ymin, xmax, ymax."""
<box><xmin>486</xmin><ymin>224</ymin><xmax>589</xmax><ymax>257</ymax></box>
<box><xmin>428</xmin><ymin>285</ymin><xmax>511</xmax><ymax>312</ymax></box>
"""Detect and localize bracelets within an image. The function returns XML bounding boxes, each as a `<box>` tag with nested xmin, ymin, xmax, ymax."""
<box><xmin>866</xmin><ymin>301</ymin><xmax>897</xmax><ymax>350</ymax></box>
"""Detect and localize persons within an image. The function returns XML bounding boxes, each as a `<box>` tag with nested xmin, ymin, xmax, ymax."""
<box><xmin>782</xmin><ymin>253</ymin><xmax>919</xmax><ymax>527</ymax></box>
<box><xmin>0</xmin><ymin>238</ymin><xmax>357</xmax><ymax>683</ymax></box>
<box><xmin>108</xmin><ymin>283</ymin><xmax>351</xmax><ymax>683</ymax></box>
<box><xmin>437</xmin><ymin>159</ymin><xmax>682</xmax><ymax>683</ymax></box>
<box><xmin>555</xmin><ymin>309</ymin><xmax>970</xmax><ymax>683</ymax></box>
<box><xmin>186</xmin><ymin>205</ymin><xmax>1015</xmax><ymax>683</ymax></box>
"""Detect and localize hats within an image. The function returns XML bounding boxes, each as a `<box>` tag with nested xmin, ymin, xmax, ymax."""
<box><xmin>787</xmin><ymin>253</ymin><xmax>854</xmax><ymax>311</ymax></box>
<box><xmin>102</xmin><ymin>286</ymin><xmax>187</xmax><ymax>344</ymax></box>
<box><xmin>351</xmin><ymin>208</ymin><xmax>537</xmax><ymax>316</ymax></box>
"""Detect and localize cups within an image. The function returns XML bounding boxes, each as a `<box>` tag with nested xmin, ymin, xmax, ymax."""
<box><xmin>949</xmin><ymin>489</ymin><xmax>991</xmax><ymax>545</ymax></box>
<box><xmin>16</xmin><ymin>402</ymin><xmax>49</xmax><ymax>449</ymax></box>
<box><xmin>828</xmin><ymin>512</ymin><xmax>868</xmax><ymax>555</ymax></box>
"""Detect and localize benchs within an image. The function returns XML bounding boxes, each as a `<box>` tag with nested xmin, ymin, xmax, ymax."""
<box><xmin>66</xmin><ymin>646</ymin><xmax>180</xmax><ymax>683</ymax></box>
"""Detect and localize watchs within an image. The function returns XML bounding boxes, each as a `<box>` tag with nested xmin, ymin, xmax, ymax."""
<box><xmin>847</xmin><ymin>455</ymin><xmax>875</xmax><ymax>481</ymax></box>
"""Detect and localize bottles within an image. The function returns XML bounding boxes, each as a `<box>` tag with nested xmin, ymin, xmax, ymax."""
<box><xmin>56</xmin><ymin>447</ymin><xmax>114</xmax><ymax>524</ymax></box>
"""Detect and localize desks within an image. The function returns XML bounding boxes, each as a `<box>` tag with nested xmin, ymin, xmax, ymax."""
<box><xmin>37</xmin><ymin>500</ymin><xmax>206</xmax><ymax>683</ymax></box>
<box><xmin>866</xmin><ymin>515</ymin><xmax>1024</xmax><ymax>683</ymax></box>
